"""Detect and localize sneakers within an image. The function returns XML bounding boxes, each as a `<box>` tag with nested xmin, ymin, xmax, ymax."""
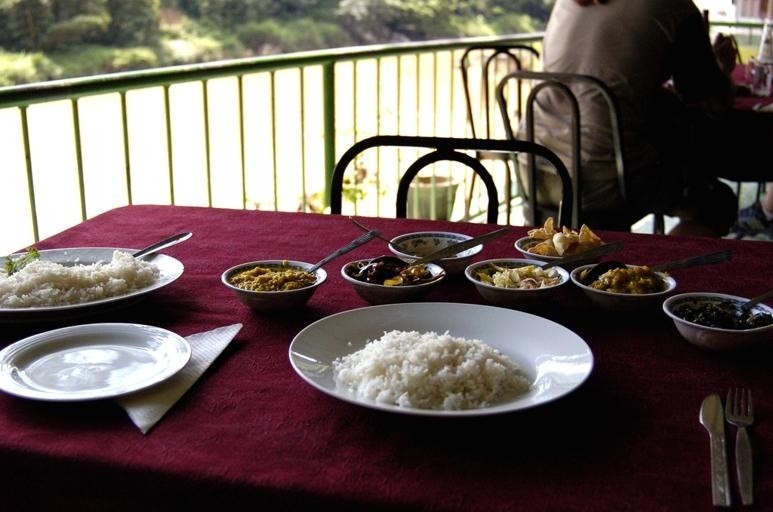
<box><xmin>731</xmin><ymin>201</ymin><xmax>773</xmax><ymax>240</ymax></box>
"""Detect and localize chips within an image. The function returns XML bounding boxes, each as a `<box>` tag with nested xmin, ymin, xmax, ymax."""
<box><xmin>527</xmin><ymin>217</ymin><xmax>601</xmax><ymax>257</ymax></box>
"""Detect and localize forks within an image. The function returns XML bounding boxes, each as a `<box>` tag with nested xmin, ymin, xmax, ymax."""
<box><xmin>725</xmin><ymin>387</ymin><xmax>755</xmax><ymax>507</ymax></box>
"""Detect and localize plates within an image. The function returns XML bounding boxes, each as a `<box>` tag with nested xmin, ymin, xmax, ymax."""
<box><xmin>0</xmin><ymin>321</ymin><xmax>191</xmax><ymax>403</ymax></box>
<box><xmin>290</xmin><ymin>302</ymin><xmax>594</xmax><ymax>419</ymax></box>
<box><xmin>0</xmin><ymin>247</ymin><xmax>185</xmax><ymax>313</ymax></box>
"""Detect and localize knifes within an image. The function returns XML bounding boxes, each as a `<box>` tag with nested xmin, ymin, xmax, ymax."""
<box><xmin>698</xmin><ymin>393</ymin><xmax>732</xmax><ymax>509</ymax></box>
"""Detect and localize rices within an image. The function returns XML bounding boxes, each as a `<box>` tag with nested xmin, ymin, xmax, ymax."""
<box><xmin>332</xmin><ymin>328</ymin><xmax>531</xmax><ymax>411</ymax></box>
<box><xmin>0</xmin><ymin>249</ymin><xmax>160</xmax><ymax>308</ymax></box>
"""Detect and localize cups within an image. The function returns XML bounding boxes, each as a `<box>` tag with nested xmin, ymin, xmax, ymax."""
<box><xmin>746</xmin><ymin>58</ymin><xmax>771</xmax><ymax>97</ymax></box>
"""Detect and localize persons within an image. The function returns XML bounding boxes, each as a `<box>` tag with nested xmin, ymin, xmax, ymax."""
<box><xmin>516</xmin><ymin>1</ymin><xmax>754</xmax><ymax>236</ymax></box>
<box><xmin>753</xmin><ymin>0</ymin><xmax>772</xmax><ymax>226</ymax></box>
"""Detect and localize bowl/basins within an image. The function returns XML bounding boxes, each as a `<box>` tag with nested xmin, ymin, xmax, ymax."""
<box><xmin>221</xmin><ymin>259</ymin><xmax>328</xmax><ymax>312</ymax></box>
<box><xmin>570</xmin><ymin>263</ymin><xmax>677</xmax><ymax>309</ymax></box>
<box><xmin>340</xmin><ymin>256</ymin><xmax>447</xmax><ymax>304</ymax></box>
<box><xmin>388</xmin><ymin>231</ymin><xmax>484</xmax><ymax>269</ymax></box>
<box><xmin>662</xmin><ymin>291</ymin><xmax>773</xmax><ymax>352</ymax></box>
<box><xmin>514</xmin><ymin>236</ymin><xmax>601</xmax><ymax>267</ymax></box>
<box><xmin>464</xmin><ymin>258</ymin><xmax>570</xmax><ymax>305</ymax></box>
